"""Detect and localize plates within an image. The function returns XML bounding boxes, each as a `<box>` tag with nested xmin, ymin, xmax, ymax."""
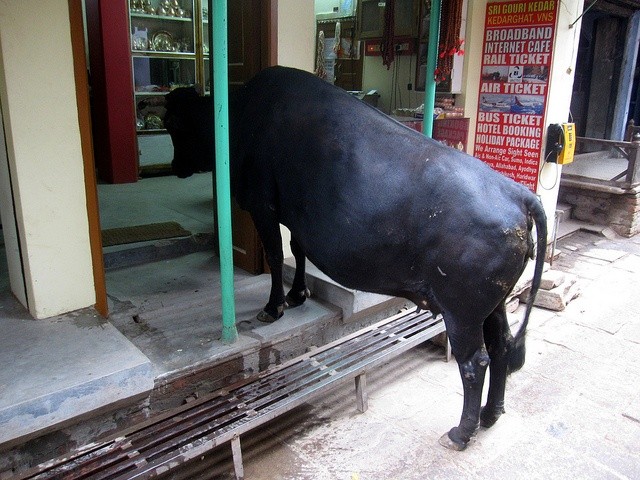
<box><xmin>151</xmin><ymin>30</ymin><xmax>174</xmax><ymax>51</ymax></box>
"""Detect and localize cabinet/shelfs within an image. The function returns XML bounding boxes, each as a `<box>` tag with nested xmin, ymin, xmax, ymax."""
<box><xmin>414</xmin><ymin>0</ymin><xmax>468</xmax><ymax>95</ymax></box>
<box><xmin>354</xmin><ymin>0</ymin><xmax>423</xmax><ymax>42</ymax></box>
<box><xmin>127</xmin><ymin>0</ymin><xmax>210</xmax><ymax>135</ymax></box>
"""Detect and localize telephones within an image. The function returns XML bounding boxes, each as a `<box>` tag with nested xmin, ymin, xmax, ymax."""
<box><xmin>538</xmin><ymin>123</ymin><xmax>575</xmax><ymax>190</ymax></box>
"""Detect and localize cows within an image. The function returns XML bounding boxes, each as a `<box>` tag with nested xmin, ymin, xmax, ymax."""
<box><xmin>162</xmin><ymin>65</ymin><xmax>548</xmax><ymax>452</ymax></box>
